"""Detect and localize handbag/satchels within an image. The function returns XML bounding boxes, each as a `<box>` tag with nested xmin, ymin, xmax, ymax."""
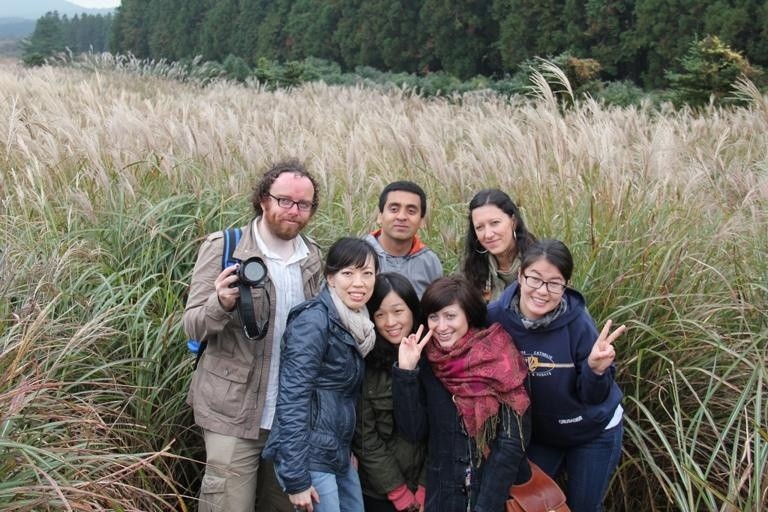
<box><xmin>482</xmin><ymin>443</ymin><xmax>572</xmax><ymax>512</ymax></box>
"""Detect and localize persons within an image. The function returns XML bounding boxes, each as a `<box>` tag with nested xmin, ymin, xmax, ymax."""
<box><xmin>485</xmin><ymin>239</ymin><xmax>625</xmax><ymax>512</ymax></box>
<box><xmin>181</xmin><ymin>163</ymin><xmax>331</xmax><ymax>511</ymax></box>
<box><xmin>261</xmin><ymin>238</ymin><xmax>381</xmax><ymax>512</ymax></box>
<box><xmin>364</xmin><ymin>181</ymin><xmax>440</xmax><ymax>300</ymax></box>
<box><xmin>420</xmin><ymin>273</ymin><xmax>529</xmax><ymax>512</ymax></box>
<box><xmin>349</xmin><ymin>273</ymin><xmax>428</xmax><ymax>512</ymax></box>
<box><xmin>463</xmin><ymin>189</ymin><xmax>537</xmax><ymax>304</ymax></box>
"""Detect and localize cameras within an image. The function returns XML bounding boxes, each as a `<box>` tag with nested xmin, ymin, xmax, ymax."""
<box><xmin>227</xmin><ymin>256</ymin><xmax>269</xmax><ymax>293</ymax></box>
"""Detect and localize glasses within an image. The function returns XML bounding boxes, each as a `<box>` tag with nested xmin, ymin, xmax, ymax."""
<box><xmin>266</xmin><ymin>192</ymin><xmax>314</xmax><ymax>212</ymax></box>
<box><xmin>523</xmin><ymin>270</ymin><xmax>566</xmax><ymax>295</ymax></box>
